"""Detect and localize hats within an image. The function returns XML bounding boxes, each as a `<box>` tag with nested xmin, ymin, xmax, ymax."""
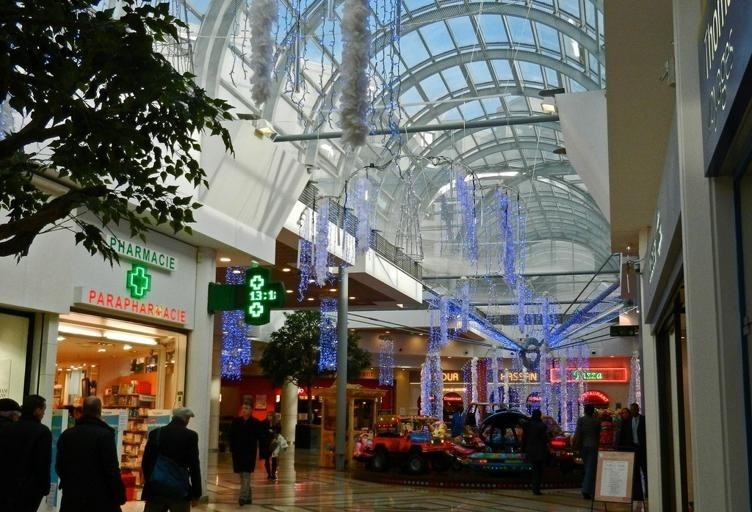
<box><xmin>173</xmin><ymin>407</ymin><xmax>194</xmax><ymax>417</ymax></box>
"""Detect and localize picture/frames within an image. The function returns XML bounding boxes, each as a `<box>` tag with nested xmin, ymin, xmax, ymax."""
<box><xmin>241</xmin><ymin>393</ymin><xmax>253</xmax><ymax>408</ymax></box>
<box><xmin>254</xmin><ymin>394</ymin><xmax>268</xmax><ymax>410</ymax></box>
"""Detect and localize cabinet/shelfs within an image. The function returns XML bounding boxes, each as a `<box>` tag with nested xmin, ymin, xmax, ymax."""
<box><xmin>102</xmin><ymin>395</ymin><xmax>155</xmax><ymax>470</ymax></box>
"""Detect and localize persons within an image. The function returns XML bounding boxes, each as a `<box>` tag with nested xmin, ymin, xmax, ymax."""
<box><xmin>229</xmin><ymin>398</ymin><xmax>269</xmax><ymax>505</ymax></box>
<box><xmin>0</xmin><ymin>396</ymin><xmax>53</xmax><ymax>512</ymax></box>
<box><xmin>257</xmin><ymin>412</ymin><xmax>281</xmax><ymax>481</ymax></box>
<box><xmin>54</xmin><ymin>396</ymin><xmax>127</xmax><ymax>512</ymax></box>
<box><xmin>522</xmin><ymin>404</ymin><xmax>645</xmax><ymax>496</ymax></box>
<box><xmin>139</xmin><ymin>408</ymin><xmax>203</xmax><ymax>512</ymax></box>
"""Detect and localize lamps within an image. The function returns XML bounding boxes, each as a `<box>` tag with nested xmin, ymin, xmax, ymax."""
<box><xmin>541</xmin><ymin>97</ymin><xmax>556</xmax><ymax>113</ymax></box>
